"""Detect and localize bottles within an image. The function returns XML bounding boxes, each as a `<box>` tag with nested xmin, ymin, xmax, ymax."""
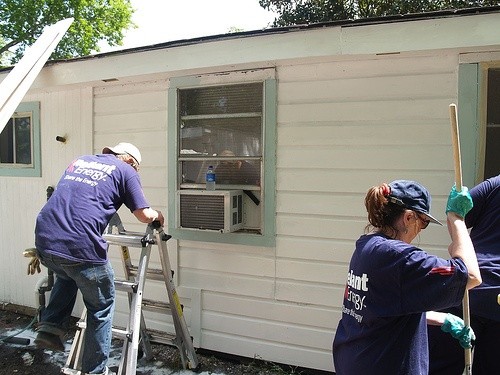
<box><xmin>205</xmin><ymin>166</ymin><xmax>215</xmax><ymax>191</ymax></box>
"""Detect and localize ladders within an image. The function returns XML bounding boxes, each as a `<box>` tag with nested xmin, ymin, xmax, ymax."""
<box><xmin>63</xmin><ymin>211</ymin><xmax>200</xmax><ymax>375</ymax></box>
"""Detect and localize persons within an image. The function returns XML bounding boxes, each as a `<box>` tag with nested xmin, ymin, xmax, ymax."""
<box><xmin>213</xmin><ymin>149</ymin><xmax>259</xmax><ymax>183</ymax></box>
<box><xmin>331</xmin><ymin>173</ymin><xmax>500</xmax><ymax>375</ymax></box>
<box><xmin>34</xmin><ymin>142</ymin><xmax>165</xmax><ymax>375</ymax></box>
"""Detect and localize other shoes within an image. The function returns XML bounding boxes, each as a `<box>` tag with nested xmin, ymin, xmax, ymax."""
<box><xmin>34</xmin><ymin>332</ymin><xmax>65</xmax><ymax>352</ymax></box>
<box><xmin>81</xmin><ymin>366</ymin><xmax>116</xmax><ymax>375</ymax></box>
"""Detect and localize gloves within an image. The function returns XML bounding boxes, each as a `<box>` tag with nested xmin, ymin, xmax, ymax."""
<box><xmin>440</xmin><ymin>313</ymin><xmax>476</xmax><ymax>349</ymax></box>
<box><xmin>445</xmin><ymin>185</ymin><xmax>473</xmax><ymax>218</ymax></box>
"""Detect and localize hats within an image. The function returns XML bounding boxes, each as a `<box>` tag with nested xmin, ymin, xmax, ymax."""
<box><xmin>385</xmin><ymin>180</ymin><xmax>443</xmax><ymax>226</ymax></box>
<box><xmin>102</xmin><ymin>142</ymin><xmax>141</xmax><ymax>165</ymax></box>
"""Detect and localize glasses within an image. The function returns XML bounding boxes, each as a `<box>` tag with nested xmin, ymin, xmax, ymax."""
<box><xmin>401</xmin><ymin>208</ymin><xmax>430</xmax><ymax>229</ymax></box>
